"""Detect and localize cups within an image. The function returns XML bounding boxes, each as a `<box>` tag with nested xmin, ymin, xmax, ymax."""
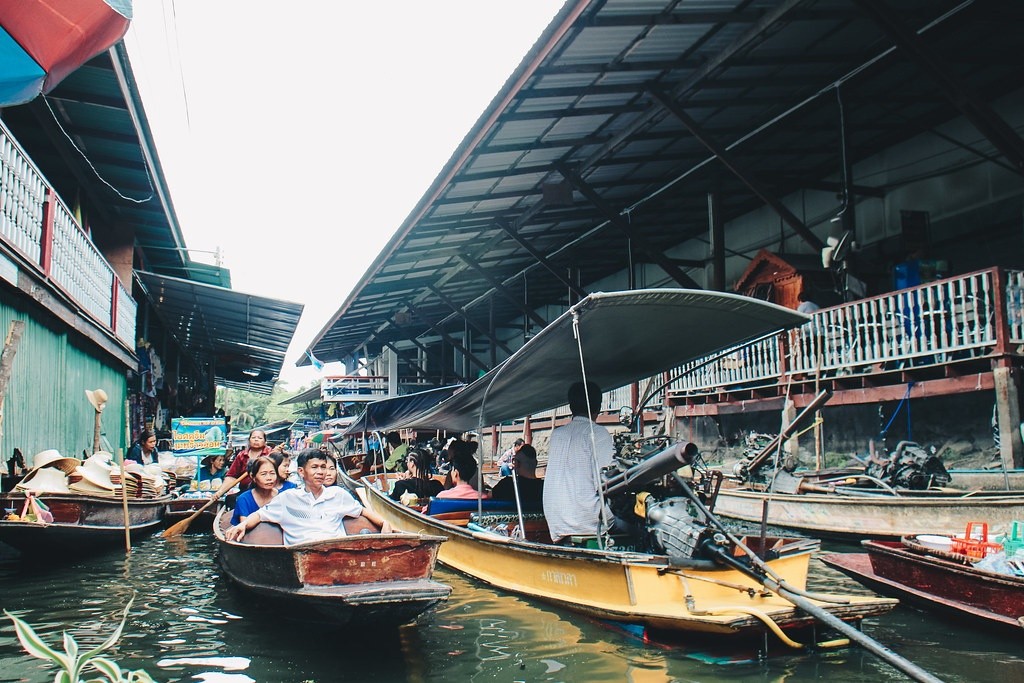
<box><xmin>5</xmin><ymin>508</ymin><xmax>17</xmax><ymax>520</ymax></box>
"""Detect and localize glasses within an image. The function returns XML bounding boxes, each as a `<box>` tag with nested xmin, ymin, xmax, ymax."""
<box><xmin>512</xmin><ymin>458</ymin><xmax>516</xmax><ymax>464</ymax></box>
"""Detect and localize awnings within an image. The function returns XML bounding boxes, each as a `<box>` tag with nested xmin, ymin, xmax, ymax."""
<box><xmin>130</xmin><ymin>267</ymin><xmax>305</xmax><ymax>397</ymax></box>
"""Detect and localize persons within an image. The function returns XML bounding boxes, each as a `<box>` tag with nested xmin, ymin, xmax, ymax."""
<box><xmin>193</xmin><ymin>429</ymin><xmax>363</xmax><ymax>531</ymax></box>
<box><xmin>127</xmin><ymin>430</ymin><xmax>158</xmax><ymax>465</ymax></box>
<box><xmin>543</xmin><ymin>383</ymin><xmax>649</xmax><ymax>551</ymax></box>
<box><xmin>355</xmin><ymin>432</ymin><xmax>545</xmax><ymax>515</ymax></box>
<box><xmin>797</xmin><ymin>291</ymin><xmax>822</xmax><ymax>328</ymax></box>
<box><xmin>226</xmin><ymin>448</ymin><xmax>392</xmax><ymax>547</ymax></box>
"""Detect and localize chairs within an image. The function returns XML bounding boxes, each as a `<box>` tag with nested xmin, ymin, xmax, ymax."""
<box><xmin>885</xmin><ymin>312</ymin><xmax>919</xmax><ymax>369</ymax></box>
<box><xmin>946</xmin><ymin>295</ymin><xmax>994</xmax><ymax>363</ymax></box>
<box><xmin>822</xmin><ymin>324</ymin><xmax>857</xmax><ymax>378</ymax></box>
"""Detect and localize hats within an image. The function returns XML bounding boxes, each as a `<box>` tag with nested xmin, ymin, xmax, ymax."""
<box><xmin>86</xmin><ymin>389</ymin><xmax>107</xmax><ymax>412</ymax></box>
<box><xmin>201</xmin><ymin>449</ymin><xmax>232</xmax><ymax>465</ymax></box>
<box><xmin>18</xmin><ymin>450</ymin><xmax>178</xmax><ymax>502</ymax></box>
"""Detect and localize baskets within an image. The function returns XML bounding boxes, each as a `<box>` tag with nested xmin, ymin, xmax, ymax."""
<box><xmin>995</xmin><ymin>521</ymin><xmax>1024</xmax><ymax>558</ymax></box>
<box><xmin>951</xmin><ymin>522</ymin><xmax>1002</xmax><ymax>557</ymax></box>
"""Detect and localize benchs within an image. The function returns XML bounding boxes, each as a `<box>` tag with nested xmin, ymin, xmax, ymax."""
<box><xmin>428</xmin><ymin>498</ymin><xmax>528</xmax><ymax>515</ymax></box>
<box><xmin>241</xmin><ymin>515</ymin><xmax>381</xmax><ymax>543</ymax></box>
<box><xmin>348</xmin><ymin>465</ymin><xmax>375</xmax><ymax>480</ymax></box>
<box><xmin>563</xmin><ymin>534</ymin><xmax>639</xmax><ymax>552</ymax></box>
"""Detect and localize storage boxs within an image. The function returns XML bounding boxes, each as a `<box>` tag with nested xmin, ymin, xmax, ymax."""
<box><xmin>950</xmin><ymin>536</ymin><xmax>1004</xmax><ymax>559</ymax></box>
<box><xmin>996</xmin><ymin>536</ymin><xmax>1024</xmax><ymax>559</ymax></box>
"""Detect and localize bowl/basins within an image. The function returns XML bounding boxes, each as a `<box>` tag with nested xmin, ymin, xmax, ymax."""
<box><xmin>399</xmin><ymin>493</ymin><xmax>418</xmax><ymax>505</ymax></box>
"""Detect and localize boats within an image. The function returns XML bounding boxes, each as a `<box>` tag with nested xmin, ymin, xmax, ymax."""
<box><xmin>694</xmin><ymin>479</ymin><xmax>1024</xmax><ymax>537</ymax></box>
<box><xmin>212</xmin><ymin>502</ymin><xmax>452</xmax><ymax>624</ymax></box>
<box><xmin>482</xmin><ymin>473</ymin><xmax>506</xmax><ymax>488</ymax></box>
<box><xmin>816</xmin><ymin>534</ymin><xmax>1024</xmax><ymax>634</ymax></box>
<box><xmin>326</xmin><ymin>383</ymin><xmax>471</xmax><ymax>494</ymax></box>
<box><xmin>355</xmin><ymin>472</ymin><xmax>901</xmax><ymax>637</ymax></box>
<box><xmin>1</xmin><ymin>490</ymin><xmax>172</xmax><ymax>553</ymax></box>
<box><xmin>164</xmin><ymin>498</ymin><xmax>224</xmax><ymax>523</ymax></box>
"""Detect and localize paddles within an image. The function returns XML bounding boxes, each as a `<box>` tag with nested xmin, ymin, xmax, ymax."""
<box><xmin>162</xmin><ymin>442</ymin><xmax>286</xmax><ymax>537</ymax></box>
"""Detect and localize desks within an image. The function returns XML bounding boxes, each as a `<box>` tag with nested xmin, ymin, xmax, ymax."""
<box><xmin>917</xmin><ymin>309</ymin><xmax>948</xmax><ymax>318</ymax></box>
<box><xmin>854</xmin><ymin>322</ymin><xmax>882</xmax><ymax>327</ymax></box>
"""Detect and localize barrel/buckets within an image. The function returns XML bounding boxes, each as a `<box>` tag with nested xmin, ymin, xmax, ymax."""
<box><xmin>916</xmin><ymin>535</ymin><xmax>957</xmax><ymax>559</ymax></box>
